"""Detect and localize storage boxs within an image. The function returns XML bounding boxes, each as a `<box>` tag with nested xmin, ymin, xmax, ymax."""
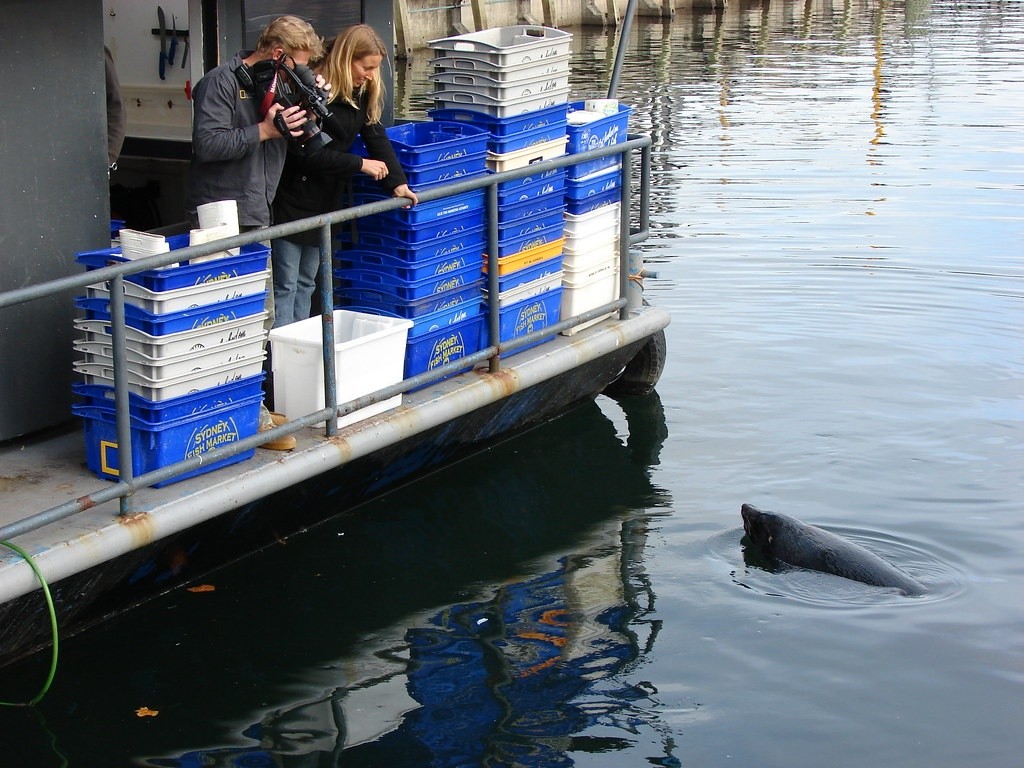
<box><xmin>268</xmin><ymin>310</ymin><xmax>414</xmax><ymax>430</ymax></box>
<box><xmin>427</xmin><ymin>23</ymin><xmax>574</xmax><ymax>361</ymax></box>
<box><xmin>70</xmin><ymin>233</ymin><xmax>271</xmax><ymax>489</ymax></box>
<box><xmin>332</xmin><ymin>121</ymin><xmax>491</xmax><ymax>394</ymax></box>
<box><xmin>560</xmin><ymin>100</ymin><xmax>634</xmax><ymax>337</ymax></box>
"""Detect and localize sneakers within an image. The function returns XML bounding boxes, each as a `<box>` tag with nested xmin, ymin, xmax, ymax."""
<box><xmin>258</xmin><ymin>411</ymin><xmax>296</xmax><ymax>451</ymax></box>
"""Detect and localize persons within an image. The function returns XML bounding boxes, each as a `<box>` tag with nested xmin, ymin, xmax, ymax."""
<box><xmin>103</xmin><ymin>43</ymin><xmax>127</xmax><ymax>180</ymax></box>
<box><xmin>181</xmin><ymin>14</ymin><xmax>334</xmax><ymax>449</ymax></box>
<box><xmin>259</xmin><ymin>24</ymin><xmax>418</xmax><ymax>415</ymax></box>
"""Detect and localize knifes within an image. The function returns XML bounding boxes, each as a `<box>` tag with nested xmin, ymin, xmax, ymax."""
<box><xmin>157</xmin><ymin>5</ymin><xmax>168</xmax><ymax>79</ymax></box>
<box><xmin>168</xmin><ymin>14</ymin><xmax>178</xmax><ymax>65</ymax></box>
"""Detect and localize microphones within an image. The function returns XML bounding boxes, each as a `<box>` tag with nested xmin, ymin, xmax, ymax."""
<box><xmin>302</xmin><ymin>86</ymin><xmax>348</xmax><ymax>142</ymax></box>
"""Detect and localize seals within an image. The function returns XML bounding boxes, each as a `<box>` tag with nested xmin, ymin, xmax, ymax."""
<box><xmin>741</xmin><ymin>498</ymin><xmax>926</xmax><ymax>598</ymax></box>
